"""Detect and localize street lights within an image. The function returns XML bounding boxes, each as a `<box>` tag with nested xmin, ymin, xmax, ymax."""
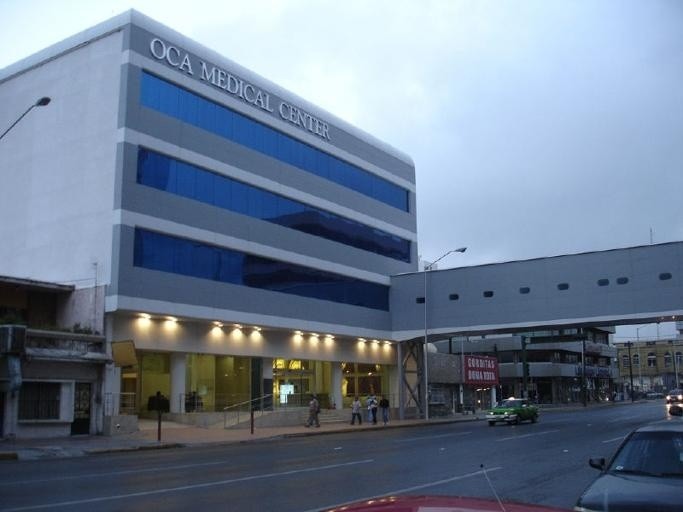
<box><xmin>424</xmin><ymin>247</ymin><xmax>468</xmax><ymax>423</ymax></box>
<box><xmin>0</xmin><ymin>97</ymin><xmax>51</xmax><ymax>142</ymax></box>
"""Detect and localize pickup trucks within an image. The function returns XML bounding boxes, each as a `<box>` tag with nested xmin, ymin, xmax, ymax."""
<box><xmin>486</xmin><ymin>397</ymin><xmax>540</xmax><ymax>428</ymax></box>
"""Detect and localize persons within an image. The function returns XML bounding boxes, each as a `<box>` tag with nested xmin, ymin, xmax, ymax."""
<box><xmin>350</xmin><ymin>397</ymin><xmax>362</xmax><ymax>425</ymax></box>
<box><xmin>190</xmin><ymin>391</ymin><xmax>198</xmax><ymax>411</ymax></box>
<box><xmin>156</xmin><ymin>392</ymin><xmax>162</xmax><ymax>400</ymax></box>
<box><xmin>380</xmin><ymin>396</ymin><xmax>391</xmax><ymax>426</ymax></box>
<box><xmin>367</xmin><ymin>394</ymin><xmax>380</xmax><ymax>425</ymax></box>
<box><xmin>306</xmin><ymin>396</ymin><xmax>320</xmax><ymax>427</ymax></box>
<box><xmin>310</xmin><ymin>393</ymin><xmax>319</xmax><ymax>425</ymax></box>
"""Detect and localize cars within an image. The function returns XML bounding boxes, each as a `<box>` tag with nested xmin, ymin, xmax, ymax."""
<box><xmin>666</xmin><ymin>389</ymin><xmax>683</xmax><ymax>404</ymax></box>
<box><xmin>573</xmin><ymin>420</ymin><xmax>683</xmax><ymax>512</ymax></box>
<box><xmin>647</xmin><ymin>391</ymin><xmax>664</xmax><ymax>399</ymax></box>
<box><xmin>319</xmin><ymin>495</ymin><xmax>575</xmax><ymax>512</ymax></box>
